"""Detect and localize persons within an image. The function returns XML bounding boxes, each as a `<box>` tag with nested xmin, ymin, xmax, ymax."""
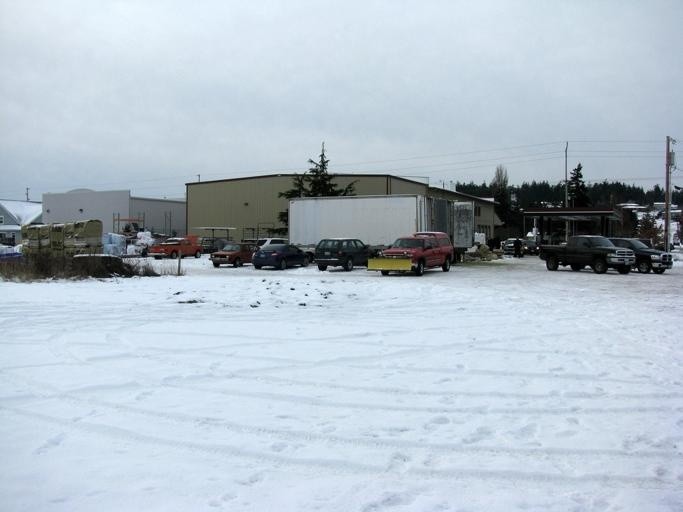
<box><xmin>512</xmin><ymin>236</ymin><xmax>521</xmax><ymax>257</ymax></box>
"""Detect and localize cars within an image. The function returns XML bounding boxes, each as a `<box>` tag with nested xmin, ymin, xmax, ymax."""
<box><xmin>253</xmin><ymin>237</ymin><xmax>309</xmax><ymax>270</ymax></box>
<box><xmin>503</xmin><ymin>238</ymin><xmax>540</xmax><ymax>257</ymax></box>
<box><xmin>208</xmin><ymin>242</ymin><xmax>257</xmax><ymax>268</ymax></box>
<box><xmin>199</xmin><ymin>237</ymin><xmax>228</xmax><ymax>255</ymax></box>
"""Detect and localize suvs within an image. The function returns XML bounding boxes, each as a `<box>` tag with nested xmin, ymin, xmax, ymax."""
<box><xmin>313</xmin><ymin>238</ymin><xmax>373</xmax><ymax>271</ymax></box>
<box><xmin>378</xmin><ymin>231</ymin><xmax>454</xmax><ymax>275</ymax></box>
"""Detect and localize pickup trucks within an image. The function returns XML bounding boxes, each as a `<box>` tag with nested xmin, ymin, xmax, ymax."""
<box><xmin>608</xmin><ymin>237</ymin><xmax>672</xmax><ymax>274</ymax></box>
<box><xmin>146</xmin><ymin>236</ymin><xmax>200</xmax><ymax>260</ymax></box>
<box><xmin>539</xmin><ymin>235</ymin><xmax>635</xmax><ymax>274</ymax></box>
